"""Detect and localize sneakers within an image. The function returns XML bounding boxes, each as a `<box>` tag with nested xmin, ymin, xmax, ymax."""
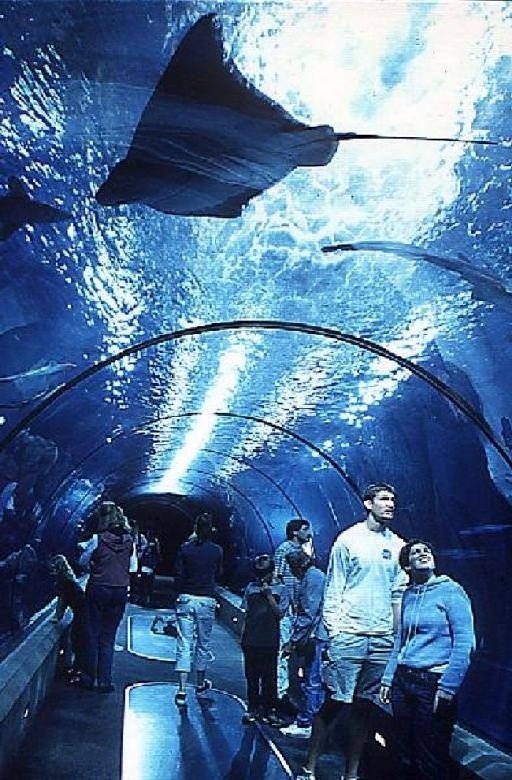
<box><xmin>241</xmin><ymin>709</ymin><xmax>312</xmax><ymax>740</ymax></box>
<box><xmin>292</xmin><ymin>765</ymin><xmax>315</xmax><ymax>780</ymax></box>
<box><xmin>68</xmin><ymin>668</ymin><xmax>116</xmax><ymax>693</ymax></box>
<box><xmin>175</xmin><ymin>679</ymin><xmax>213</xmax><ymax>707</ymax></box>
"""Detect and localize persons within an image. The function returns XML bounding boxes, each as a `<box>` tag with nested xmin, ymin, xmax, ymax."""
<box><xmin>378</xmin><ymin>539</ymin><xmax>476</xmax><ymax>779</ymax></box>
<box><xmin>47</xmin><ymin>489</ymin><xmax>162</xmax><ymax>695</ymax></box>
<box><xmin>239</xmin><ymin>519</ymin><xmax>327</xmax><ymax>739</ymax></box>
<box><xmin>174</xmin><ymin>513</ymin><xmax>225</xmax><ymax>706</ymax></box>
<box><xmin>294</xmin><ymin>481</ymin><xmax>408</xmax><ymax>780</ymax></box>
